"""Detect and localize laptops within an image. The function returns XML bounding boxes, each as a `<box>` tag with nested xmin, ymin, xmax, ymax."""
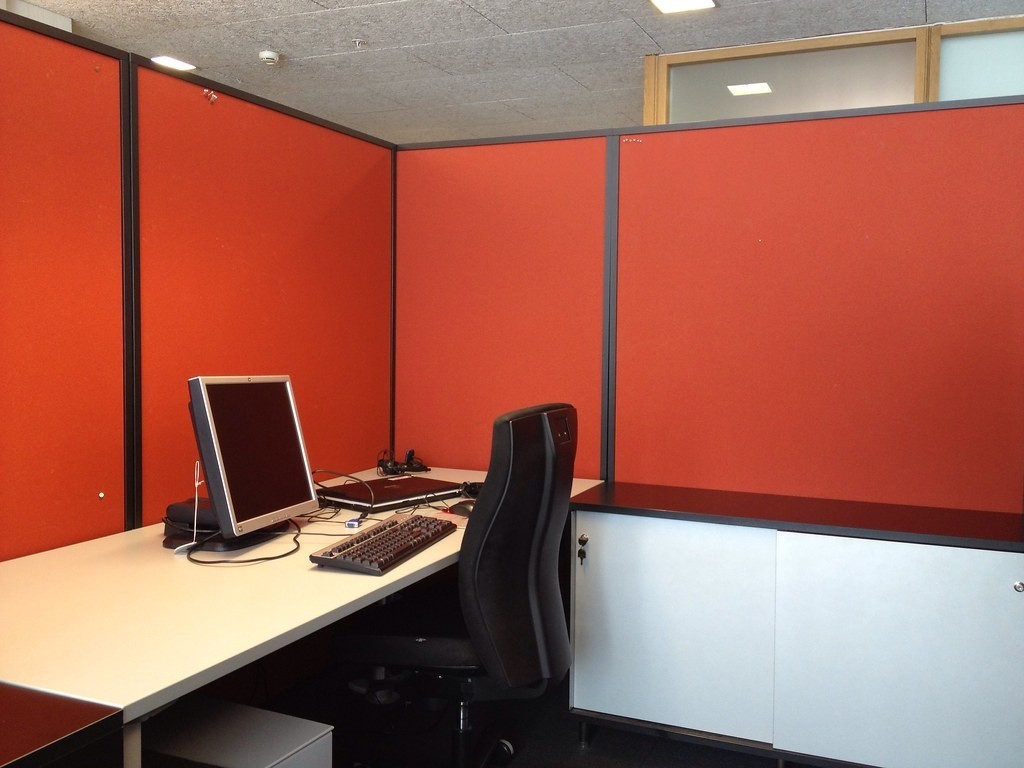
<box><xmin>316</xmin><ymin>475</ymin><xmax>463</xmax><ymax>513</ymax></box>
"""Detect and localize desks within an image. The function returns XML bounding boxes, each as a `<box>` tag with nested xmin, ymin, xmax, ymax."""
<box><xmin>0</xmin><ymin>465</ymin><xmax>608</xmax><ymax>768</ymax></box>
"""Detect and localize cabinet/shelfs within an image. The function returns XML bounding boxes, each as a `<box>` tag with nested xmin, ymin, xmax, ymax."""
<box><xmin>571</xmin><ymin>480</ymin><xmax>1024</xmax><ymax>768</ymax></box>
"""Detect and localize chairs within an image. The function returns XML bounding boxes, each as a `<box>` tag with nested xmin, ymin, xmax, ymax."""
<box><xmin>330</xmin><ymin>403</ymin><xmax>578</xmax><ymax>768</ymax></box>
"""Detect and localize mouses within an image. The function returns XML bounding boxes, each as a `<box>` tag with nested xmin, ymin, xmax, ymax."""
<box><xmin>449</xmin><ymin>501</ymin><xmax>475</xmax><ymax>518</ymax></box>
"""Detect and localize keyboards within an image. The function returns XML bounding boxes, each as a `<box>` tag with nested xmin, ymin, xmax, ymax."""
<box><xmin>308</xmin><ymin>513</ymin><xmax>457</xmax><ymax>575</ymax></box>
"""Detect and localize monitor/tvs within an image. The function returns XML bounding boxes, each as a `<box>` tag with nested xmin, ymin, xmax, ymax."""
<box><xmin>187</xmin><ymin>374</ymin><xmax>319</xmax><ymax>552</ymax></box>
